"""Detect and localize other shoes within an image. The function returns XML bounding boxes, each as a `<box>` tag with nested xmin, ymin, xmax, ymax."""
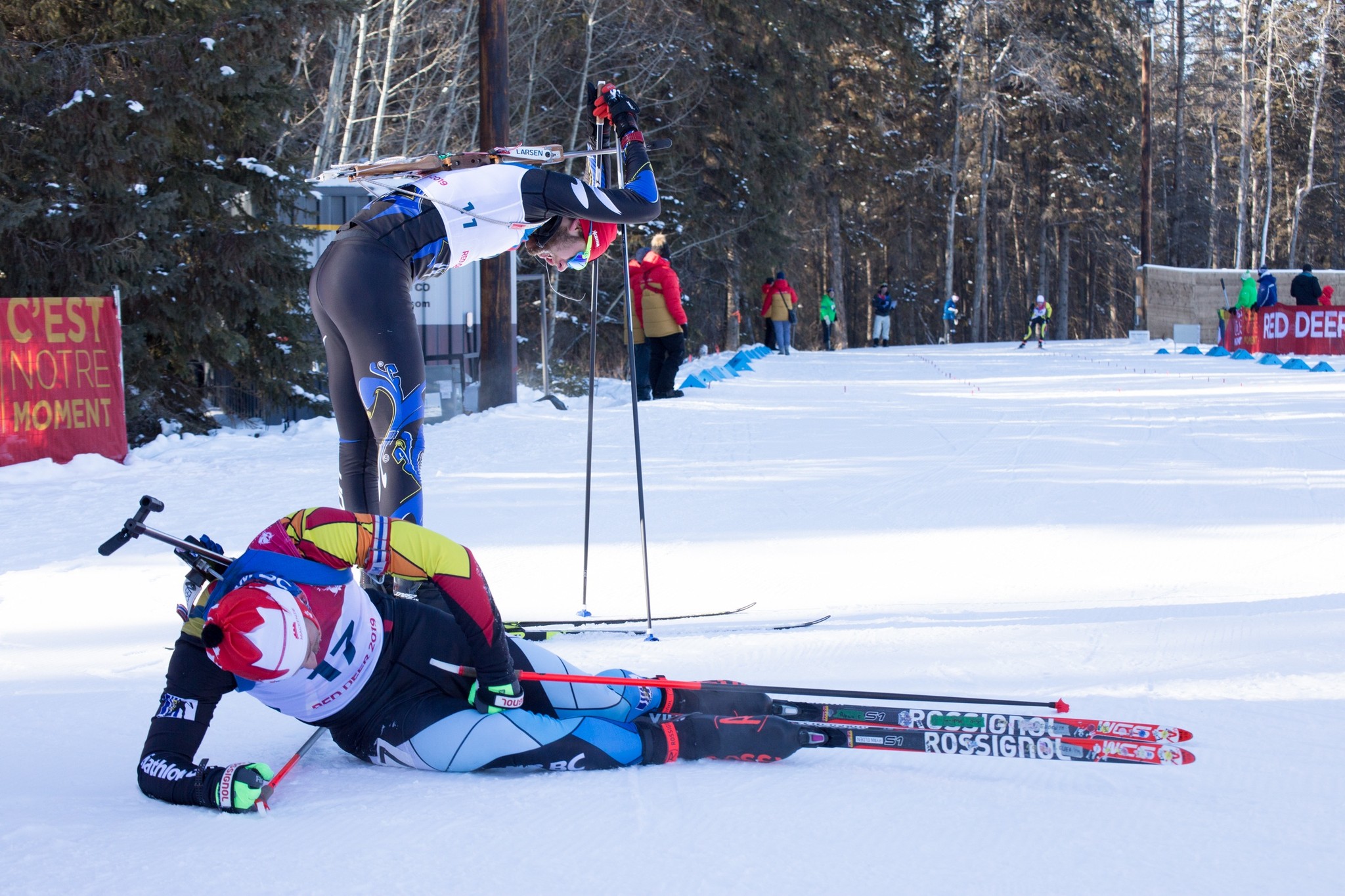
<box><xmin>874</xmin><ymin>339</ymin><xmax>879</xmax><ymax>347</ymax></box>
<box><xmin>1038</xmin><ymin>342</ymin><xmax>1042</xmax><ymax>349</ymax></box>
<box><xmin>685</xmin><ymin>710</ymin><xmax>794</xmax><ymax>763</ymax></box>
<box><xmin>653</xmin><ymin>389</ymin><xmax>684</xmax><ymax>399</ymax></box>
<box><xmin>669</xmin><ymin>679</ymin><xmax>771</xmax><ymax>716</ymax></box>
<box><xmin>883</xmin><ymin>339</ymin><xmax>888</xmax><ymax>347</ymax></box>
<box><xmin>1020</xmin><ymin>344</ymin><xmax>1025</xmax><ymax>348</ymax></box>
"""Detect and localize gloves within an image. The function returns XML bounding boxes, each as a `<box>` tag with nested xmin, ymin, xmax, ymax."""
<box><xmin>210</xmin><ymin>764</ymin><xmax>273</xmax><ymax>813</ymax></box>
<box><xmin>881</xmin><ymin>301</ymin><xmax>886</xmax><ymax>305</ymax></box>
<box><xmin>467</xmin><ymin>681</ymin><xmax>522</xmax><ymax>716</ymax></box>
<box><xmin>824</xmin><ymin>316</ymin><xmax>831</xmax><ymax>326</ymax></box>
<box><xmin>681</xmin><ymin>324</ymin><xmax>688</xmax><ymax>339</ymax></box>
<box><xmin>594</xmin><ymin>82</ymin><xmax>639</xmax><ymax>138</ymax></box>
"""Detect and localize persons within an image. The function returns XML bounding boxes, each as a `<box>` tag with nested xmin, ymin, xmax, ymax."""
<box><xmin>759</xmin><ymin>272</ymin><xmax>803</xmax><ymax>355</ymax></box>
<box><xmin>1228</xmin><ymin>271</ymin><xmax>1257</xmax><ymax>315</ymax></box>
<box><xmin>623</xmin><ymin>233</ymin><xmax>689</xmax><ymax>401</ymax></box>
<box><xmin>1019</xmin><ymin>295</ymin><xmax>1053</xmax><ymax>349</ymax></box>
<box><xmin>1317</xmin><ymin>286</ymin><xmax>1334</xmax><ymax>306</ymax></box>
<box><xmin>311</xmin><ymin>83</ymin><xmax>661</xmax><ymax>527</ymax></box>
<box><xmin>1290</xmin><ymin>264</ymin><xmax>1322</xmax><ymax>306</ymax></box>
<box><xmin>1250</xmin><ymin>265</ymin><xmax>1277</xmax><ymax>312</ymax></box>
<box><xmin>137</xmin><ymin>508</ymin><xmax>805</xmax><ymax>813</ymax></box>
<box><xmin>819</xmin><ymin>289</ymin><xmax>837</xmax><ymax>352</ymax></box>
<box><xmin>871</xmin><ymin>282</ymin><xmax>893</xmax><ymax>347</ymax></box>
<box><xmin>942</xmin><ymin>294</ymin><xmax>959</xmax><ymax>343</ymax></box>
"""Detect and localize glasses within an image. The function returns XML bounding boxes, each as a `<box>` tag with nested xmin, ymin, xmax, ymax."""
<box><xmin>567</xmin><ymin>222</ymin><xmax>592</xmax><ymax>270</ymax></box>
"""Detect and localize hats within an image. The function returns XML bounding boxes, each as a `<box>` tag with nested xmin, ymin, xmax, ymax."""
<box><xmin>580</xmin><ymin>217</ymin><xmax>617</xmax><ymax>263</ymax></box>
<box><xmin>651</xmin><ymin>234</ymin><xmax>671</xmax><ymax>258</ymax></box>
<box><xmin>1241</xmin><ymin>271</ymin><xmax>1250</xmax><ymax>281</ymax></box>
<box><xmin>1037</xmin><ymin>295</ymin><xmax>1044</xmax><ymax>303</ymax></box>
<box><xmin>636</xmin><ymin>248</ymin><xmax>651</xmax><ymax>263</ymax></box>
<box><xmin>1257</xmin><ymin>264</ymin><xmax>1267</xmax><ymax>275</ymax></box>
<box><xmin>201</xmin><ymin>583</ymin><xmax>308</xmax><ymax>683</ymax></box>
<box><xmin>880</xmin><ymin>282</ymin><xmax>888</xmax><ymax>289</ymax></box>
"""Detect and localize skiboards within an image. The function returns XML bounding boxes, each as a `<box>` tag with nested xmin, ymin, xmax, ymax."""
<box><xmin>1013</xmin><ymin>347</ymin><xmax>1047</xmax><ymax>351</ymax></box>
<box><xmin>501</xmin><ymin>602</ymin><xmax>832</xmax><ymax>641</ymax></box>
<box><xmin>771</xmin><ymin>700</ymin><xmax>1195</xmax><ymax>765</ymax></box>
